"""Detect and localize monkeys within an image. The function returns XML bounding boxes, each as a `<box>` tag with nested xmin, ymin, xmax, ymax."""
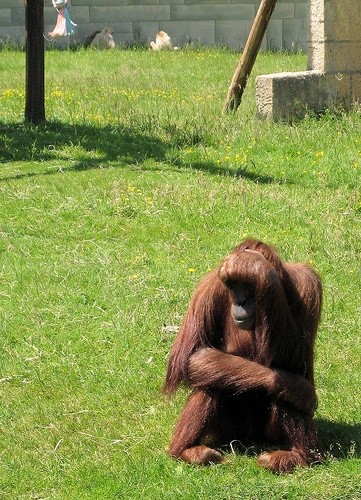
<box><xmin>150</xmin><ymin>30</ymin><xmax>179</xmax><ymax>52</ymax></box>
<box><xmin>89</xmin><ymin>26</ymin><xmax>115</xmax><ymax>49</ymax></box>
